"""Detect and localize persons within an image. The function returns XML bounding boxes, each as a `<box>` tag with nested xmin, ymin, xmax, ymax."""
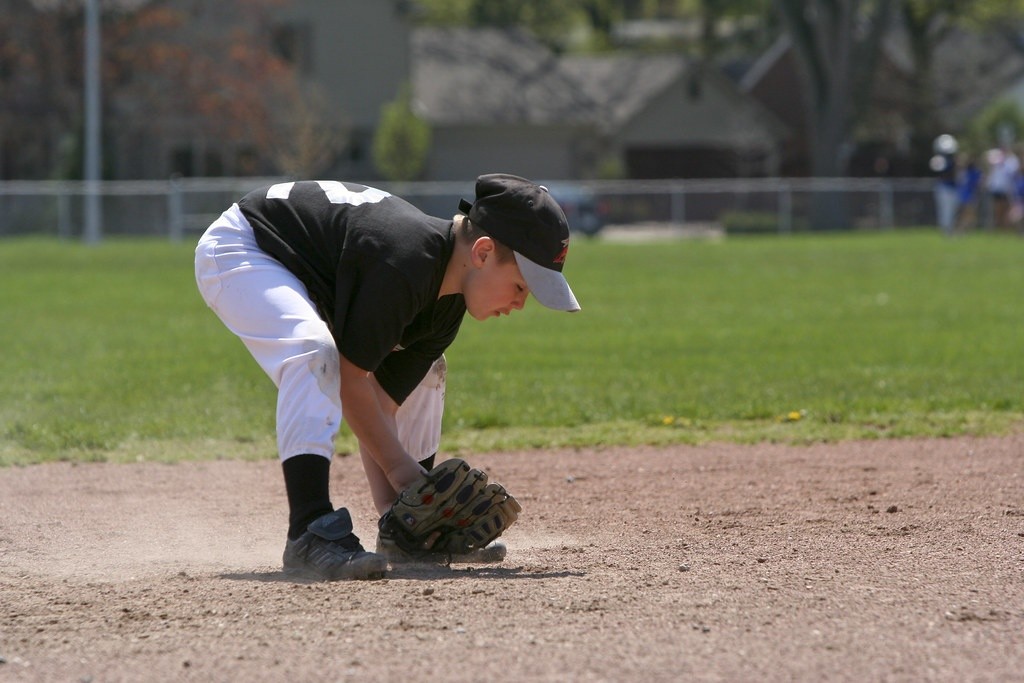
<box><xmin>928</xmin><ymin>135</ymin><xmax>1020</xmax><ymax>231</ymax></box>
<box><xmin>194</xmin><ymin>173</ymin><xmax>571</xmax><ymax>579</ymax></box>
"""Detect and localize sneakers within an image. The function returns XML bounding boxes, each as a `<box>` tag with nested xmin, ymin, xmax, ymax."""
<box><xmin>282</xmin><ymin>508</ymin><xmax>386</xmax><ymax>581</ymax></box>
<box><xmin>375</xmin><ymin>508</ymin><xmax>506</xmax><ymax>563</ymax></box>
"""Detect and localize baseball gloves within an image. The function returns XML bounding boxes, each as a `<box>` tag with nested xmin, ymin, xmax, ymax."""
<box><xmin>376</xmin><ymin>456</ymin><xmax>524</xmax><ymax>570</ymax></box>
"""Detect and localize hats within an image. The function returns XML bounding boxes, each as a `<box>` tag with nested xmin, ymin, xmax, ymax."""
<box><xmin>458</xmin><ymin>173</ymin><xmax>580</xmax><ymax>312</ymax></box>
